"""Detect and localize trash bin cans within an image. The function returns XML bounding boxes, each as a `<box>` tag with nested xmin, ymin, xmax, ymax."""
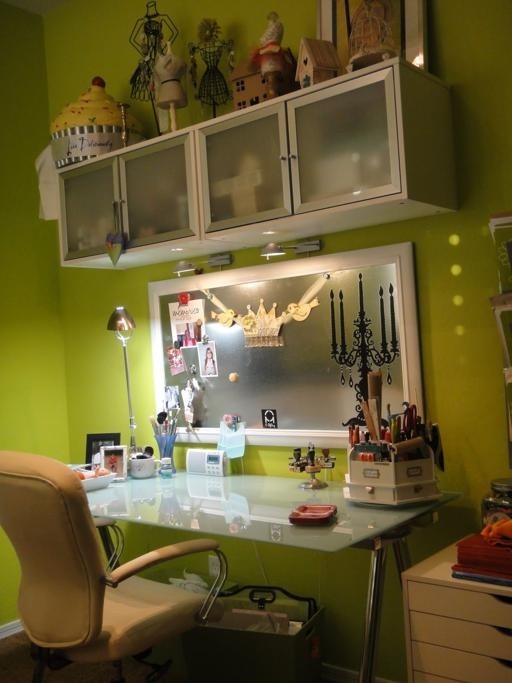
<box><xmin>199</xmin><ymin>604</ymin><xmax>324</xmax><ymax>682</ymax></box>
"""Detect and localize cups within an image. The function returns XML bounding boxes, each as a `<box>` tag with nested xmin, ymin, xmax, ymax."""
<box><xmin>153</xmin><ymin>434</ymin><xmax>178</xmax><ymax>474</ymax></box>
<box><xmin>130</xmin><ymin>456</ymin><xmax>163</xmax><ymax>479</ymax></box>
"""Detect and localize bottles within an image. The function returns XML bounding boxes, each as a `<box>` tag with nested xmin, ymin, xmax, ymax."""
<box><xmin>159</xmin><ymin>457</ymin><xmax>173</xmax><ymax>477</ymax></box>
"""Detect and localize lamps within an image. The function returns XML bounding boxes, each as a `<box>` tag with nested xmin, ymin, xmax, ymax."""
<box><xmin>172</xmin><ymin>253</ymin><xmax>231</xmax><ymax>274</ymax></box>
<box><xmin>106</xmin><ymin>306</ymin><xmax>143</xmax><ymax>469</ymax></box>
<box><xmin>259</xmin><ymin>239</ymin><xmax>321</xmax><ymax>257</ymax></box>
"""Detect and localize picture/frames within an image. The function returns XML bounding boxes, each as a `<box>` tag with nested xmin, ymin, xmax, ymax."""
<box><xmin>315</xmin><ymin>0</ymin><xmax>428</xmax><ymax>77</ymax></box>
<box><xmin>85</xmin><ymin>432</ymin><xmax>127</xmax><ymax>482</ymax></box>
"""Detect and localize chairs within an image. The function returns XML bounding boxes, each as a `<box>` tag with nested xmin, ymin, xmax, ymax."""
<box><xmin>0</xmin><ymin>449</ymin><xmax>229</xmax><ymax>682</ymax></box>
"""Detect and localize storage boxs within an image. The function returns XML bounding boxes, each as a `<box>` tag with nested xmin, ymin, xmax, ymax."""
<box><xmin>180</xmin><ymin>595</ymin><xmax>324</xmax><ymax>682</ymax></box>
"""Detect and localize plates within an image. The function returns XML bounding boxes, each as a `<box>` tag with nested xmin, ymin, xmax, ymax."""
<box><xmin>75</xmin><ymin>468</ymin><xmax>118</xmax><ymax>492</ymax></box>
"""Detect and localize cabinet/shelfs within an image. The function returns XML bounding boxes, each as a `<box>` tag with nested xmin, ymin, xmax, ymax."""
<box><xmin>55</xmin><ymin>56</ymin><xmax>458</xmax><ymax>270</ymax></box>
<box><xmin>401</xmin><ymin>531</ymin><xmax>512</xmax><ymax>682</ymax></box>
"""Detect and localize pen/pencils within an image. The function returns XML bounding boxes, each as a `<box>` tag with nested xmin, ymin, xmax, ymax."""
<box><xmin>150</xmin><ymin>416</ymin><xmax>178</xmax><ymax>458</ymax></box>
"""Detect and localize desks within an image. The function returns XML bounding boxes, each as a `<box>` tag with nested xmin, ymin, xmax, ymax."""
<box><xmin>84</xmin><ymin>467</ymin><xmax>464</xmax><ymax>682</ymax></box>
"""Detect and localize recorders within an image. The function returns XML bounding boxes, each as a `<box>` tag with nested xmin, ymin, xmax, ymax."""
<box><xmin>186</xmin><ymin>475</ymin><xmax>234</xmax><ymax>501</ymax></box>
<box><xmin>185</xmin><ymin>448</ymin><xmax>228</xmax><ymax>478</ymax></box>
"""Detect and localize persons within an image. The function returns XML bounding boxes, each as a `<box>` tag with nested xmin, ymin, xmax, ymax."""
<box><xmin>256</xmin><ymin>11</ymin><xmax>285</xmax><ymax>75</ymax></box>
<box><xmin>181</xmin><ymin>379</ymin><xmax>196</xmax><ymax>432</ymax></box>
<box><xmin>203</xmin><ymin>347</ymin><xmax>217</xmax><ymax>376</ymax></box>
<box><xmin>109</xmin><ymin>452</ymin><xmax>119</xmax><ymax>474</ymax></box>
<box><xmin>154</xmin><ymin>51</ymin><xmax>189</xmax><ymax>109</ymax></box>
<box><xmin>182</xmin><ymin>330</ymin><xmax>198</xmax><ymax>348</ymax></box>
